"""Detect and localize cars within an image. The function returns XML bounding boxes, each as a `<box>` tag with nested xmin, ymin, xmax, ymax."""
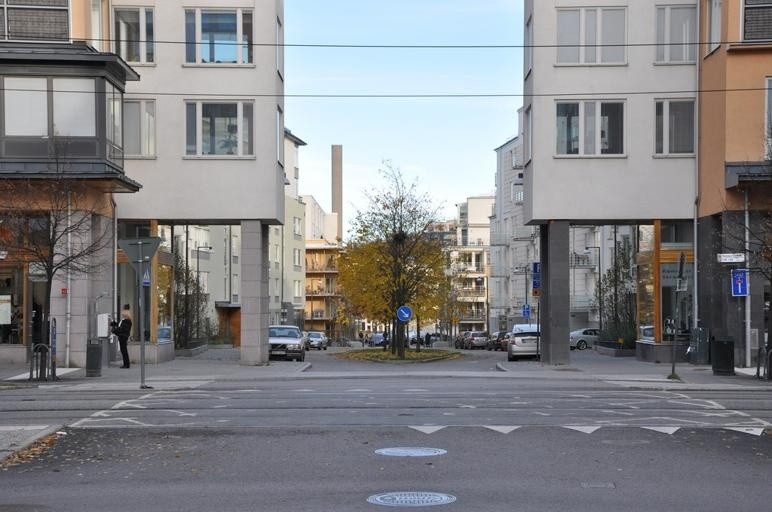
<box><xmin>267</xmin><ymin>325</ymin><xmax>329</xmax><ymax>363</ymax></box>
<box><xmin>455</xmin><ymin>323</ymin><xmax>541</xmax><ymax>363</ymax></box>
<box><xmin>569</xmin><ymin>328</ymin><xmax>605</xmax><ymax>350</ymax></box>
<box><xmin>369</xmin><ymin>333</ymin><xmax>452</xmax><ymax>348</ymax></box>
<box><xmin>639</xmin><ymin>325</ymin><xmax>656</xmax><ymax>344</ymax></box>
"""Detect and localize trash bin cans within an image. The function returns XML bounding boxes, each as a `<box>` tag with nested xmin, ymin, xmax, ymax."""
<box><xmin>85</xmin><ymin>338</ymin><xmax>103</xmax><ymax>377</ymax></box>
<box><xmin>710</xmin><ymin>340</ymin><xmax>735</xmax><ymax>376</ymax></box>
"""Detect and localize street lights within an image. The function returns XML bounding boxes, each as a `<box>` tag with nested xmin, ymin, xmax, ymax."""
<box><xmin>195</xmin><ymin>244</ymin><xmax>214</xmax><ymax>342</ymax></box>
<box><xmin>477</xmin><ymin>275</ymin><xmax>490</xmax><ymax>331</ymax></box>
<box><xmin>310</xmin><ymin>278</ymin><xmax>321</xmax><ymax>327</ymax></box>
<box><xmin>584</xmin><ymin>246</ymin><xmax>601</xmax><ymax>334</ymax></box>
<box><xmin>514</xmin><ymin>265</ymin><xmax>527</xmax><ymax>323</ymax></box>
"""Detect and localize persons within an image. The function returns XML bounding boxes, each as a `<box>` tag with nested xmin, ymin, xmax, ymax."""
<box><xmin>109</xmin><ymin>310</ymin><xmax>132</xmax><ymax>368</ymax></box>
